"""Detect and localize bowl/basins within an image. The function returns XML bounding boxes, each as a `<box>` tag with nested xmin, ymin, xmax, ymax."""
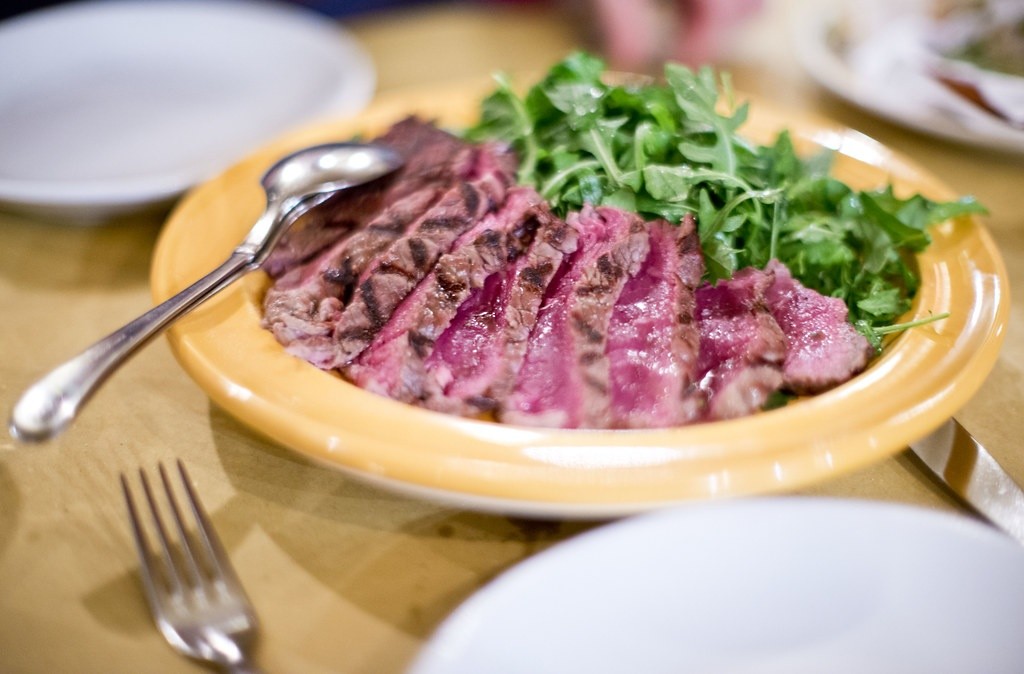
<box><xmin>149</xmin><ymin>88</ymin><xmax>1012</xmax><ymax>519</ymax></box>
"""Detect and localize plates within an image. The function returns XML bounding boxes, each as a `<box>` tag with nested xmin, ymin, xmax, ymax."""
<box><xmin>795</xmin><ymin>1</ymin><xmax>1024</xmax><ymax>156</ymax></box>
<box><xmin>0</xmin><ymin>0</ymin><xmax>380</xmax><ymax>223</ymax></box>
<box><xmin>407</xmin><ymin>497</ymin><xmax>1024</xmax><ymax>673</ymax></box>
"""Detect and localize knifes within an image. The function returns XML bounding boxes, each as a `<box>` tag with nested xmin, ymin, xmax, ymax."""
<box><xmin>910</xmin><ymin>417</ymin><xmax>1023</xmax><ymax>538</ymax></box>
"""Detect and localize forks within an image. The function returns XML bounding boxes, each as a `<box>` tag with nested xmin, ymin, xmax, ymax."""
<box><xmin>116</xmin><ymin>457</ymin><xmax>262</xmax><ymax>674</ymax></box>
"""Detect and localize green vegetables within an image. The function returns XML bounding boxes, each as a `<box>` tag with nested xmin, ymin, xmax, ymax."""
<box><xmin>462</xmin><ymin>47</ymin><xmax>993</xmax><ymax>409</ymax></box>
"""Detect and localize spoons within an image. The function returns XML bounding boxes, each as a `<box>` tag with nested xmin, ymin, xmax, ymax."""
<box><xmin>9</xmin><ymin>140</ymin><xmax>404</xmax><ymax>445</ymax></box>
